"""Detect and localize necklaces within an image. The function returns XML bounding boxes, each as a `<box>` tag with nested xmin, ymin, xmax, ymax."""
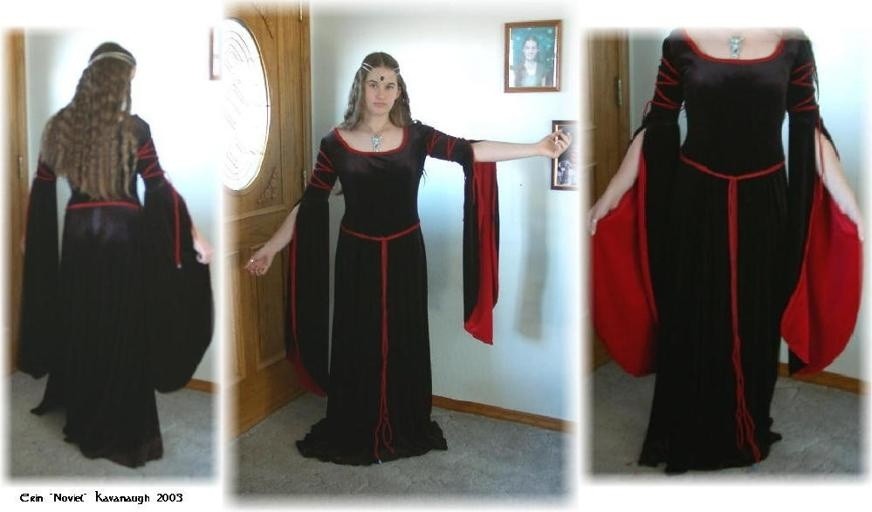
<box><xmin>360</xmin><ymin>117</ymin><xmax>391</xmax><ymax>153</ymax></box>
<box><xmin>721</xmin><ymin>28</ymin><xmax>753</xmax><ymax>58</ymax></box>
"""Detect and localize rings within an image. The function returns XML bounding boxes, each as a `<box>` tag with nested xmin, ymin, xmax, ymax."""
<box><xmin>591</xmin><ymin>218</ymin><xmax>597</xmax><ymax>223</ymax></box>
<box><xmin>248</xmin><ymin>256</ymin><xmax>256</xmax><ymax>264</ymax></box>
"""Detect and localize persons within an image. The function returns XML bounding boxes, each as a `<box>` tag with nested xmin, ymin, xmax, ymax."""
<box><xmin>239</xmin><ymin>50</ymin><xmax>569</xmax><ymax>468</ymax></box>
<box><xmin>512</xmin><ymin>36</ymin><xmax>546</xmax><ymax>87</ymax></box>
<box><xmin>590</xmin><ymin>28</ymin><xmax>864</xmax><ymax>473</ymax></box>
<box><xmin>12</xmin><ymin>41</ymin><xmax>213</xmax><ymax>470</ymax></box>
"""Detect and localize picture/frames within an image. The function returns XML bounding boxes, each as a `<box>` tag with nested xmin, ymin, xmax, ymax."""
<box><xmin>550</xmin><ymin>120</ymin><xmax>579</xmax><ymax>191</ymax></box>
<box><xmin>504</xmin><ymin>20</ymin><xmax>561</xmax><ymax>93</ymax></box>
